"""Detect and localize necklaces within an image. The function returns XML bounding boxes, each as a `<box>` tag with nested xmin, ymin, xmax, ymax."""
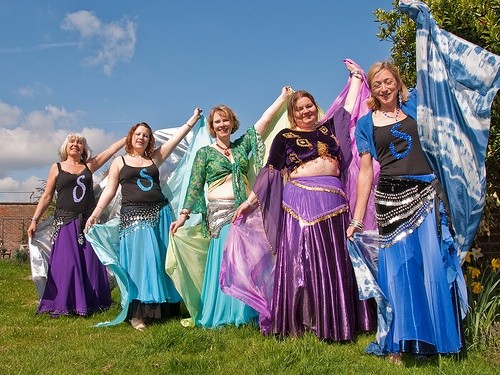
<box><xmin>382</xmin><ymin>108</ymin><xmax>399</xmax><ymax>121</ymax></box>
<box><xmin>216</xmin><ymin>142</ymin><xmax>230</xmax><ymax>156</ymax></box>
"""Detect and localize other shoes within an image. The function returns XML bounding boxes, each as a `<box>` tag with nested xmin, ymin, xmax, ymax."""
<box><xmin>128</xmin><ymin>318</ymin><xmax>147</xmax><ymax>332</ymax></box>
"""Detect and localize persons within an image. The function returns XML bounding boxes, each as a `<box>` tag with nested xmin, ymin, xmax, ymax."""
<box><xmin>27</xmin><ymin>132</ymin><xmax>128</xmax><ymax>320</ymax></box>
<box><xmin>345</xmin><ymin>0</ymin><xmax>500</xmax><ymax>365</ymax></box>
<box><xmin>163</xmin><ymin>86</ymin><xmax>325</xmax><ymax>330</ymax></box>
<box><xmin>86</xmin><ymin>108</ymin><xmax>216</xmax><ymax>333</ymax></box>
<box><xmin>218</xmin><ymin>58</ymin><xmax>381</xmax><ymax>343</ymax></box>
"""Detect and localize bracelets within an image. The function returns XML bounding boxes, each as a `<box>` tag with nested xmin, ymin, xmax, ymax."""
<box><xmin>179</xmin><ymin>211</ymin><xmax>191</xmax><ymax>220</ymax></box>
<box><xmin>348</xmin><ymin>219</ymin><xmax>364</xmax><ymax>231</ymax></box>
<box><xmin>92</xmin><ymin>216</ymin><xmax>99</xmax><ymax>219</ymax></box>
<box><xmin>186</xmin><ymin>122</ymin><xmax>192</xmax><ymax>128</ymax></box>
<box><xmin>244</xmin><ymin>200</ymin><xmax>253</xmax><ymax>206</ymax></box>
<box><xmin>31</xmin><ymin>216</ymin><xmax>39</xmax><ymax>222</ymax></box>
<box><xmin>351</xmin><ymin>71</ymin><xmax>362</xmax><ymax>79</ymax></box>
